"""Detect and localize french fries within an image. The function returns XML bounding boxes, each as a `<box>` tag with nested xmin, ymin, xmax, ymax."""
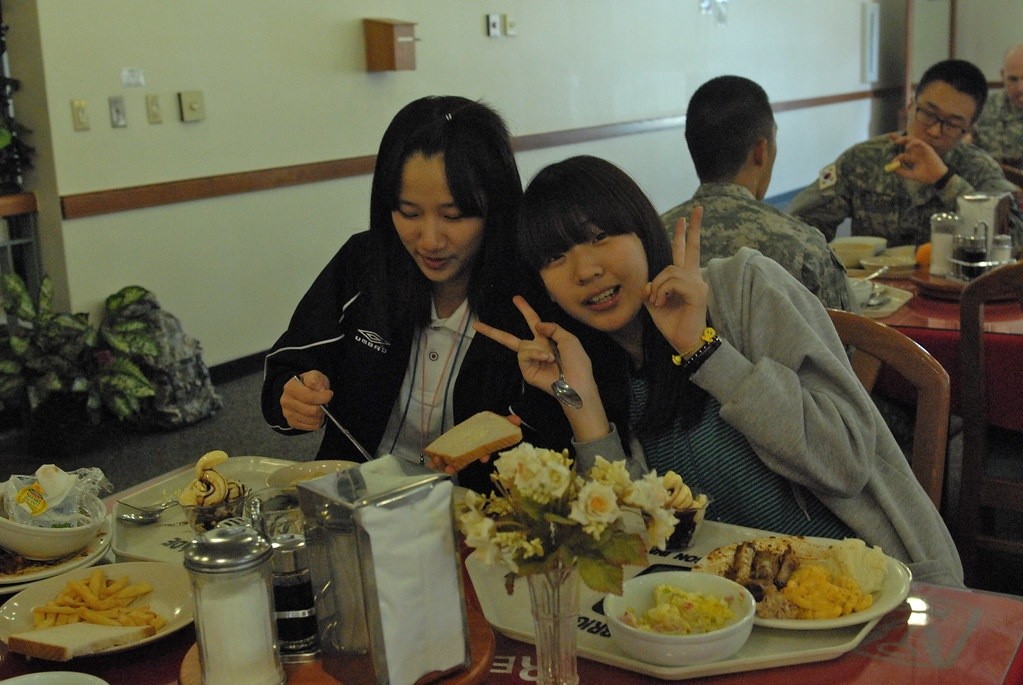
<box><xmin>884</xmin><ymin>161</ymin><xmax>900</xmax><ymax>173</ymax></box>
<box><xmin>789</xmin><ymin>565</ymin><xmax>872</xmax><ymax>620</ymax></box>
<box><xmin>32</xmin><ymin>568</ymin><xmax>167</xmax><ymax>629</ymax></box>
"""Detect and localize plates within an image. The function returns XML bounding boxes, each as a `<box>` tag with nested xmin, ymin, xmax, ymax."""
<box><xmin>265</xmin><ymin>460</ymin><xmax>362</xmax><ymax>488</ymax></box>
<box><xmin>881</xmin><ymin>245</ymin><xmax>916</xmax><ymax>258</ymax></box>
<box><xmin>1</xmin><ymin>560</ymin><xmax>194</xmax><ymax>655</ymax></box>
<box><xmin>0</xmin><ymin>513</ymin><xmax>113</xmax><ymax>594</ymax></box>
<box><xmin>688</xmin><ymin>535</ymin><xmax>912</xmax><ymax>630</ymax></box>
<box><xmin>0</xmin><ymin>671</ymin><xmax>110</xmax><ymax>685</ymax></box>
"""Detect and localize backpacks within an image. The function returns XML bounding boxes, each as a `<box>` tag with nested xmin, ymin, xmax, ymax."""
<box><xmin>97</xmin><ymin>298</ymin><xmax>224</xmax><ymax>431</ymax></box>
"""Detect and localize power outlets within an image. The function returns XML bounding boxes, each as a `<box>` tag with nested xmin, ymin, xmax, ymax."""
<box><xmin>488</xmin><ymin>14</ymin><xmax>501</xmax><ymax>37</ymax></box>
<box><xmin>146</xmin><ymin>93</ymin><xmax>164</xmax><ymax>125</ymax></box>
<box><xmin>108</xmin><ymin>95</ymin><xmax>127</xmax><ymax>129</ymax></box>
<box><xmin>71</xmin><ymin>99</ymin><xmax>91</xmax><ymax>132</ymax></box>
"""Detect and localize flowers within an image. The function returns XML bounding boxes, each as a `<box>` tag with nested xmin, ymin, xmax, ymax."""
<box><xmin>460</xmin><ymin>439</ymin><xmax>677</xmax><ymax>601</ymax></box>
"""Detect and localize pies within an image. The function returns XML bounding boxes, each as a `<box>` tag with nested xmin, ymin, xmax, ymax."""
<box><xmin>705</xmin><ymin>535</ymin><xmax>825</xmax><ymax>576</ymax></box>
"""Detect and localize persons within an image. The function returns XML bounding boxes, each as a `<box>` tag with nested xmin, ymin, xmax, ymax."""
<box><xmin>969</xmin><ymin>43</ymin><xmax>1023</xmax><ymax>189</ymax></box>
<box><xmin>261</xmin><ymin>97</ymin><xmax>551</xmax><ymax>494</ymax></box>
<box><xmin>660</xmin><ymin>74</ymin><xmax>864</xmax><ymax>381</ymax></box>
<box><xmin>471</xmin><ymin>154</ymin><xmax>965</xmax><ymax>591</ymax></box>
<box><xmin>786</xmin><ymin>58</ymin><xmax>1023</xmax><ymax>294</ymax></box>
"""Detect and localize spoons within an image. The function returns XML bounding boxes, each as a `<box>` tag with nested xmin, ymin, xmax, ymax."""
<box><xmin>547</xmin><ymin>335</ymin><xmax>583</xmax><ymax>409</ymax></box>
<box><xmin>116</xmin><ymin>501</ymin><xmax>179</xmax><ymax>523</ymax></box>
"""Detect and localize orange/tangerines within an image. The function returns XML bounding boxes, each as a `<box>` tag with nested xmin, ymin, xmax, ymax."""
<box><xmin>916</xmin><ymin>243</ymin><xmax>933</xmax><ymax>267</ymax></box>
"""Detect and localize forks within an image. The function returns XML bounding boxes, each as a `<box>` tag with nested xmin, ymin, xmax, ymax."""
<box><xmin>117</xmin><ymin>500</ymin><xmax>177</xmax><ymax>512</ymax></box>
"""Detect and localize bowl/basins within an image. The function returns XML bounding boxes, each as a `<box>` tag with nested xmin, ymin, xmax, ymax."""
<box><xmin>848</xmin><ymin>277</ymin><xmax>872</xmax><ymax>304</ymax></box>
<box><xmin>602</xmin><ymin>571</ymin><xmax>758</xmax><ymax>666</ymax></box>
<box><xmin>0</xmin><ymin>477</ymin><xmax>108</xmax><ymax>560</ymax></box>
<box><xmin>833</xmin><ymin>236</ymin><xmax>887</xmax><ymax>268</ymax></box>
<box><xmin>860</xmin><ymin>256</ymin><xmax>916</xmax><ymax>279</ymax></box>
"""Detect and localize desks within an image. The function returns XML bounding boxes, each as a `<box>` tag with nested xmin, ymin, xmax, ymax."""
<box><xmin>0</xmin><ymin>458</ymin><xmax>1023</xmax><ymax>685</ymax></box>
<box><xmin>838</xmin><ymin>264</ymin><xmax>1023</xmax><ymax>578</ymax></box>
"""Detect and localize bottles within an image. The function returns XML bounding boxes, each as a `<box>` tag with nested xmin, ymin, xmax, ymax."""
<box><xmin>267</xmin><ymin>533</ymin><xmax>324</xmax><ymax>665</ymax></box>
<box><xmin>952</xmin><ymin>231</ymin><xmax>1014</xmax><ymax>282</ymax></box>
<box><xmin>928</xmin><ymin>211</ymin><xmax>964</xmax><ymax>276</ymax></box>
<box><xmin>185</xmin><ymin>527</ymin><xmax>288</xmax><ymax>685</ymax></box>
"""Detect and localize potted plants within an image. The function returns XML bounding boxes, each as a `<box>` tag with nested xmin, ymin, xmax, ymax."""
<box><xmin>0</xmin><ymin>270</ymin><xmax>159</xmax><ymax>461</ymax></box>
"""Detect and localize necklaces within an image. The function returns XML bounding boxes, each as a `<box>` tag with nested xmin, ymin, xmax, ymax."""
<box><xmin>419</xmin><ymin>304</ymin><xmax>469</xmax><ymax>465</ymax></box>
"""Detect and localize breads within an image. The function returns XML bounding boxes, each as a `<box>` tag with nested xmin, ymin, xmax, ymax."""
<box><xmin>8</xmin><ymin>621</ymin><xmax>156</xmax><ymax>661</ymax></box>
<box><xmin>423</xmin><ymin>411</ymin><xmax>523</xmax><ymax>469</ymax></box>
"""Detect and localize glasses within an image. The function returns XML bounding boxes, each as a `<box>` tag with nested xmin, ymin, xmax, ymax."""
<box><xmin>913</xmin><ymin>98</ymin><xmax>969</xmax><ymax>139</ymax></box>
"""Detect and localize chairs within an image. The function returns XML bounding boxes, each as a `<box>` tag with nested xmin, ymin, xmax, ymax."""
<box><xmin>825</xmin><ymin>258</ymin><xmax>1023</xmax><ymax>598</ymax></box>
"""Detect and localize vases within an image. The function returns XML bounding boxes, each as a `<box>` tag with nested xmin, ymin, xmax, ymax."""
<box><xmin>522</xmin><ymin>556</ymin><xmax>584</xmax><ymax>685</ymax></box>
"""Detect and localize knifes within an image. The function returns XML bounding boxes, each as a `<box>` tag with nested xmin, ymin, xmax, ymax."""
<box><xmin>294</xmin><ymin>374</ymin><xmax>373</xmax><ymax>461</ymax></box>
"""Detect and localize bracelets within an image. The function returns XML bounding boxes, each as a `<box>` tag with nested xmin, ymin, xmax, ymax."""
<box><xmin>671</xmin><ymin>328</ymin><xmax>722</xmax><ymax>379</ymax></box>
<box><xmin>935</xmin><ymin>167</ymin><xmax>955</xmax><ymax>190</ymax></box>
